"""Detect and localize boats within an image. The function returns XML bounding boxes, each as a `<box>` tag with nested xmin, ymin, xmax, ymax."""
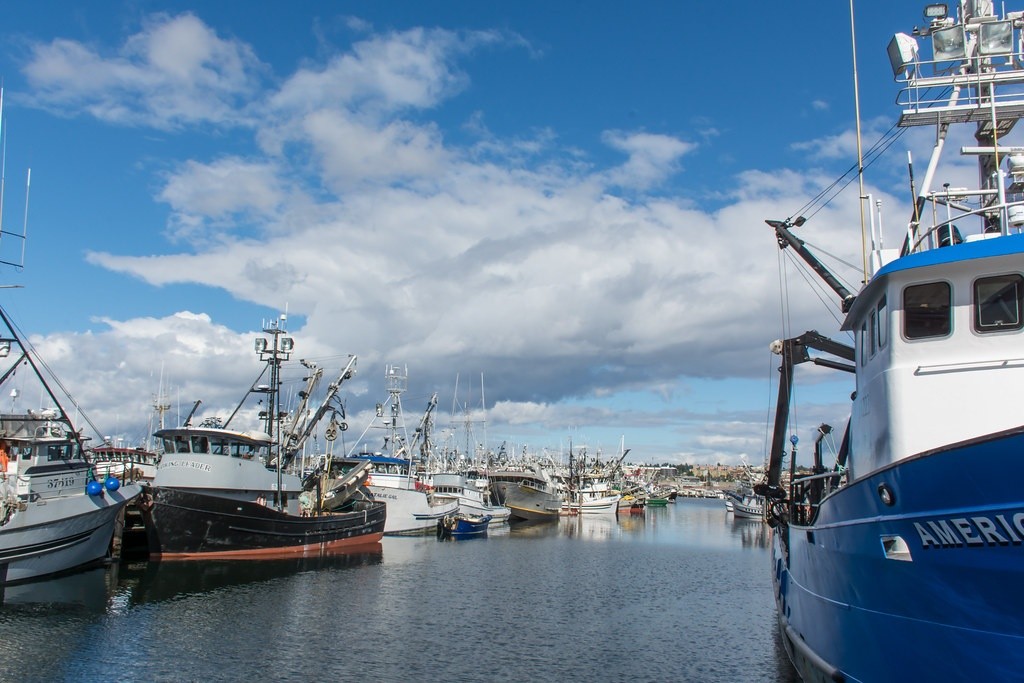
<box><xmin>715</xmin><ymin>459</ymin><xmax>810</xmax><ymax>519</ymax></box>
<box><xmin>0</xmin><ymin>86</ymin><xmax>676</xmax><ymax>609</ymax></box>
<box><xmin>750</xmin><ymin>0</ymin><xmax>1024</xmax><ymax>683</ymax></box>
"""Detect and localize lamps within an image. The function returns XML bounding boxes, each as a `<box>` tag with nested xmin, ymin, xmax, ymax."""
<box><xmin>977</xmin><ymin>20</ymin><xmax>1014</xmax><ymax>55</ymax></box>
<box><xmin>930</xmin><ymin>23</ymin><xmax>972</xmax><ymax>74</ymax></box>
<box><xmin>886</xmin><ymin>32</ymin><xmax>923</xmax><ymax>79</ymax></box>
<box><xmin>281</xmin><ymin>338</ymin><xmax>294</xmax><ymax>350</ymax></box>
<box><xmin>376</xmin><ymin>403</ymin><xmax>383</xmax><ymax>412</ymax></box>
<box><xmin>391</xmin><ymin>404</ymin><xmax>399</xmax><ymax>412</ymax></box>
<box><xmin>255</xmin><ymin>337</ymin><xmax>267</xmax><ymax>352</ymax></box>
